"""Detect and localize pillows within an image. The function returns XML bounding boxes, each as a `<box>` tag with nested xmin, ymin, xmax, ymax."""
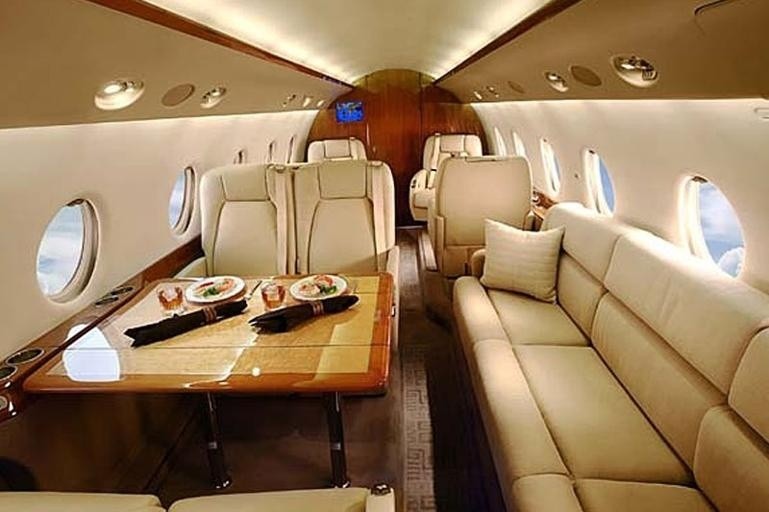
<box><xmin>482</xmin><ymin>218</ymin><xmax>567</xmax><ymax>305</ymax></box>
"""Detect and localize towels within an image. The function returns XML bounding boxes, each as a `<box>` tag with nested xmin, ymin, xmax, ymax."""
<box><xmin>123</xmin><ymin>299</ymin><xmax>246</xmax><ymax>348</ymax></box>
<box><xmin>248</xmin><ymin>295</ymin><xmax>358</xmax><ymax>332</ymax></box>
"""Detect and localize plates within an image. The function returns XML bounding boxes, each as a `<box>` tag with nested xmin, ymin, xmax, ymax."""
<box><xmin>288</xmin><ymin>272</ymin><xmax>347</xmax><ymax>301</ymax></box>
<box><xmin>185</xmin><ymin>275</ymin><xmax>245</xmax><ymax>303</ymax></box>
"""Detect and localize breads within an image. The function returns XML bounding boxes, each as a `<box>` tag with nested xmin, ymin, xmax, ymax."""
<box><xmin>314</xmin><ymin>274</ymin><xmax>332</xmax><ymax>289</ymax></box>
<box><xmin>215</xmin><ymin>279</ymin><xmax>233</xmax><ymax>292</ymax></box>
<box><xmin>299</xmin><ymin>284</ymin><xmax>320</xmax><ymax>297</ymax></box>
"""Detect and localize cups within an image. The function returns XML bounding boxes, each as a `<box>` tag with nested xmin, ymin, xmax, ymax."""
<box><xmin>261</xmin><ymin>277</ymin><xmax>284</xmax><ymax>309</ymax></box>
<box><xmin>156</xmin><ymin>288</ymin><xmax>185</xmax><ymax>317</ymax></box>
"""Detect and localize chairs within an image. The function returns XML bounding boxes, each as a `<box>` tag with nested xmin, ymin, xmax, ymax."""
<box><xmin>410</xmin><ymin>133</ymin><xmax>483</xmax><ymax>224</ymax></box>
<box><xmin>291</xmin><ymin>162</ymin><xmax>401</xmax><ymax>352</ymax></box>
<box><xmin>197</xmin><ymin>163</ymin><xmax>290</xmax><ymax>279</ymax></box>
<box><xmin>308</xmin><ymin>137</ymin><xmax>366</xmax><ymax>161</ymax></box>
<box><xmin>0</xmin><ymin>487</ymin><xmax>167</xmax><ymax>512</ymax></box>
<box><xmin>168</xmin><ymin>484</ymin><xmax>396</xmax><ymax>512</ymax></box>
<box><xmin>426</xmin><ymin>152</ymin><xmax>533</xmax><ymax>276</ymax></box>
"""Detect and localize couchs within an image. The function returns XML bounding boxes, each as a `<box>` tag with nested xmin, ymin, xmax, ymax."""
<box><xmin>453</xmin><ymin>201</ymin><xmax>769</xmax><ymax>512</ymax></box>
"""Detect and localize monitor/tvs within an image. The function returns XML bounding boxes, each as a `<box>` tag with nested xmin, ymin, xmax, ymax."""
<box><xmin>336</xmin><ymin>101</ymin><xmax>364</xmax><ymax>122</ymax></box>
